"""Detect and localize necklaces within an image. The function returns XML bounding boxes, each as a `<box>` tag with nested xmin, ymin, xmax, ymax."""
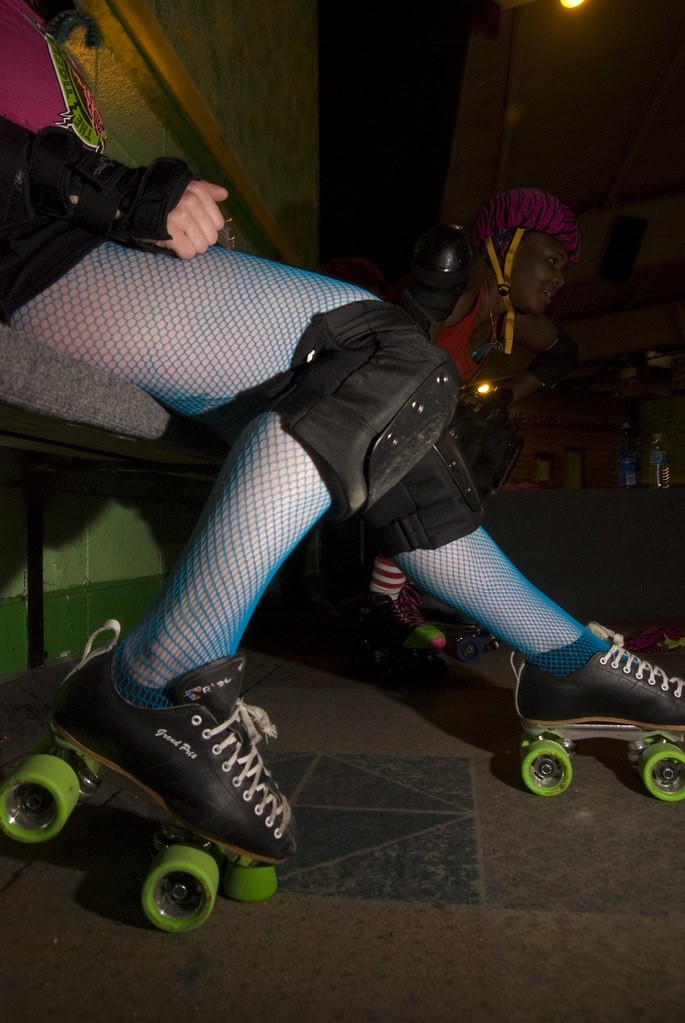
<box><xmin>485</xmin><ymin>279</ymin><xmax>504</xmax><ymax>351</ymax></box>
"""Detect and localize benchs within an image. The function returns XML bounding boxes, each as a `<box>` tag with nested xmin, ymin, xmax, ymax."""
<box><xmin>0</xmin><ymin>324</ymin><xmax>232</xmax><ymax>667</ymax></box>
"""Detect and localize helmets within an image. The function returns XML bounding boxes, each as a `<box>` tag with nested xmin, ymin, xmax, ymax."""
<box><xmin>472</xmin><ymin>185</ymin><xmax>580</xmax><ymax>264</ymax></box>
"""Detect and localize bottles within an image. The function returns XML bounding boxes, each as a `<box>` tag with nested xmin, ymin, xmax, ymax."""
<box><xmin>648</xmin><ymin>433</ymin><xmax>673</xmax><ymax>489</ymax></box>
<box><xmin>615</xmin><ymin>422</ymin><xmax>640</xmax><ymax>488</ymax></box>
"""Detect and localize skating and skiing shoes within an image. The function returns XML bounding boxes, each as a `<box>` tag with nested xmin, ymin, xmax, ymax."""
<box><xmin>509</xmin><ymin>619</ymin><xmax>685</xmax><ymax>802</ymax></box>
<box><xmin>458</xmin><ymin>614</ymin><xmax>503</xmax><ymax>662</ymax></box>
<box><xmin>353</xmin><ymin>590</ymin><xmax>447</xmax><ymax>686</ymax></box>
<box><xmin>0</xmin><ymin>617</ymin><xmax>302</xmax><ymax>930</ymax></box>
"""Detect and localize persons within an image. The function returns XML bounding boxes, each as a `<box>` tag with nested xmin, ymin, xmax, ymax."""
<box><xmin>348</xmin><ymin>186</ymin><xmax>583</xmax><ymax>679</ymax></box>
<box><xmin>0</xmin><ymin>0</ymin><xmax>684</xmax><ymax>932</ymax></box>
